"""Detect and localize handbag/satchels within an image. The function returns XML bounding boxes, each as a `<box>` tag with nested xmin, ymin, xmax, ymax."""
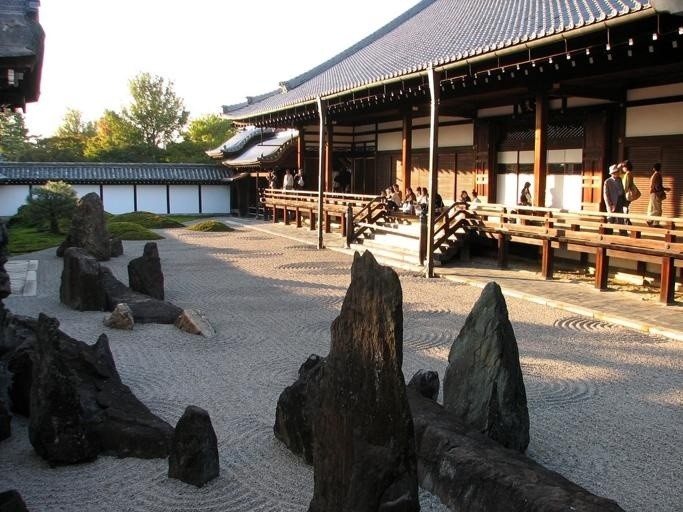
<box><xmin>656</xmin><ymin>192</ymin><xmax>666</xmax><ymax>201</ymax></box>
<box><xmin>625</xmin><ymin>186</ymin><xmax>641</xmax><ymax>202</ymax></box>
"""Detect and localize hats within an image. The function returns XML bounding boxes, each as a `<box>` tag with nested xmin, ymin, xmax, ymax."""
<box><xmin>609</xmin><ymin>164</ymin><xmax>618</xmax><ymax>174</ymax></box>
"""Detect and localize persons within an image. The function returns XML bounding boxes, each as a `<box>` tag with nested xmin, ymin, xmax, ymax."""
<box><xmin>603</xmin><ymin>159</ymin><xmax>634</xmax><ymax>235</ymax></box>
<box><xmin>384</xmin><ymin>184</ymin><xmax>444</xmax><ymax>216</ymax></box>
<box><xmin>645</xmin><ymin>163</ymin><xmax>671</xmax><ymax>228</ymax></box>
<box><xmin>519</xmin><ymin>181</ymin><xmax>532</xmax><ymax>224</ymax></box>
<box><xmin>266</xmin><ymin>165</ymin><xmax>351</xmax><ymax>193</ymax></box>
<box><xmin>461</xmin><ymin>190</ymin><xmax>471</xmax><ymax>210</ymax></box>
<box><xmin>471</xmin><ymin>191</ymin><xmax>482</xmax><ymax>210</ymax></box>
